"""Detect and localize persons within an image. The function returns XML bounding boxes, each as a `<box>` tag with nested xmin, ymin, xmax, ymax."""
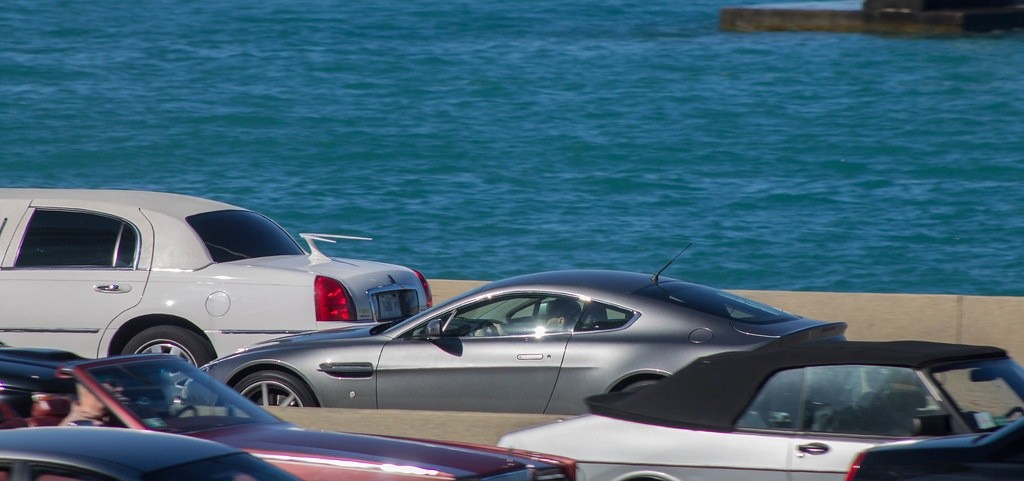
<box><xmin>58</xmin><ymin>365</ymin><xmax>129</xmax><ymax>428</ymax></box>
<box><xmin>839</xmin><ymin>366</ymin><xmax>952</xmax><ymax>436</ymax></box>
<box><xmin>474</xmin><ymin>298</ymin><xmax>580</xmax><ymax>337</ymax></box>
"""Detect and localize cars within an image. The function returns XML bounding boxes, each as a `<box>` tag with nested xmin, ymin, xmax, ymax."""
<box><xmin>850</xmin><ymin>415</ymin><xmax>1024</xmax><ymax>481</ymax></box>
<box><xmin>0</xmin><ymin>344</ymin><xmax>581</xmax><ymax>480</ymax></box>
<box><xmin>0</xmin><ymin>187</ymin><xmax>434</xmax><ymax>396</ymax></box>
<box><xmin>0</xmin><ymin>428</ymin><xmax>301</xmax><ymax>480</ymax></box>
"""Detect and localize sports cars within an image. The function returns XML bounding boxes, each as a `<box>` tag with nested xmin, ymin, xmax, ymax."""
<box><xmin>182</xmin><ymin>268</ymin><xmax>849</xmax><ymax>418</ymax></box>
<box><xmin>496</xmin><ymin>341</ymin><xmax>1024</xmax><ymax>481</ymax></box>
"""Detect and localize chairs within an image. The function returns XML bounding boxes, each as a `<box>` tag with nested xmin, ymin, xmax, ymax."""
<box><xmin>24</xmin><ymin>398</ymin><xmax>71</xmax><ymax>427</ymax></box>
<box><xmin>808</xmin><ymin>379</ymin><xmax>855</xmax><ymax>432</ymax></box>
<box><xmin>858</xmin><ymin>392</ymin><xmax>895</xmax><ymax>431</ymax></box>
